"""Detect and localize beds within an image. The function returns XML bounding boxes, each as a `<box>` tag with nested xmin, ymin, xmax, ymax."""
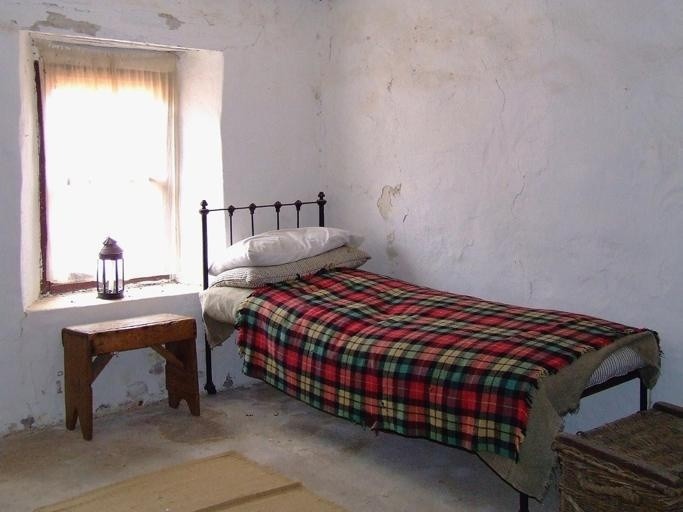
<box><xmin>199</xmin><ymin>191</ymin><xmax>665</xmax><ymax>512</ymax></box>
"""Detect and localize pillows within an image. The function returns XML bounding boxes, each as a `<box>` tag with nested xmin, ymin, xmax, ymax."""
<box><xmin>210</xmin><ymin>246</ymin><xmax>370</xmax><ymax>288</ymax></box>
<box><xmin>208</xmin><ymin>227</ymin><xmax>365</xmax><ymax>276</ymax></box>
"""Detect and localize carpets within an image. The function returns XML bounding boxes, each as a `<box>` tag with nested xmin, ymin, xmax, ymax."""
<box><xmin>33</xmin><ymin>451</ymin><xmax>346</xmax><ymax>512</ymax></box>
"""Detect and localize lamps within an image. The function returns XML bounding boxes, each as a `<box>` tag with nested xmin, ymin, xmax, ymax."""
<box><xmin>96</xmin><ymin>235</ymin><xmax>125</xmax><ymax>299</ymax></box>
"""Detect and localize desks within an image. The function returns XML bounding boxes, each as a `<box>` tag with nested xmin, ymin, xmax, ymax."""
<box><xmin>62</xmin><ymin>313</ymin><xmax>200</xmax><ymax>441</ymax></box>
